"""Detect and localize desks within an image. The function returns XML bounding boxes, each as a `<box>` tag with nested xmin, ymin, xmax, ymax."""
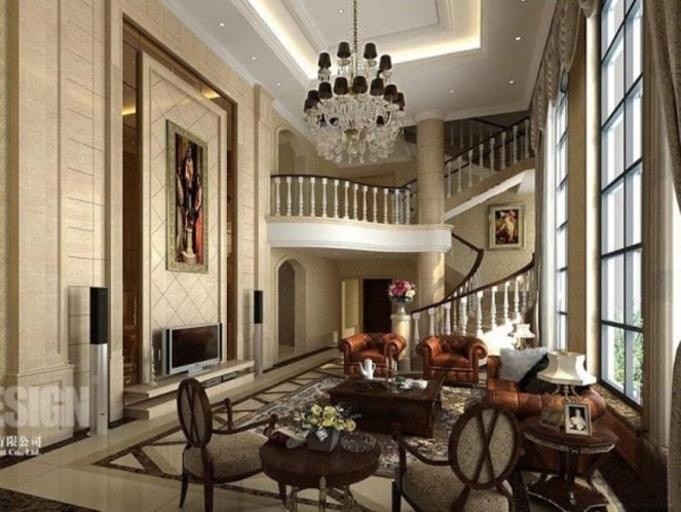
<box><xmin>261</xmin><ymin>434</ymin><xmax>382</xmax><ymax>511</ymax></box>
<box><xmin>522</xmin><ymin>418</ymin><xmax>618</xmax><ymax>511</ymax></box>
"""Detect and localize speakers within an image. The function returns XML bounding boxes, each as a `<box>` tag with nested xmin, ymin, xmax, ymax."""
<box><xmin>253</xmin><ymin>290</ymin><xmax>265</xmax><ymax>379</ymax></box>
<box><xmin>87</xmin><ymin>286</ymin><xmax>110</xmax><ymax>437</ymax></box>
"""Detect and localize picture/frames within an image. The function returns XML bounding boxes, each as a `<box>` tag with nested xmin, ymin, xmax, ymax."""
<box><xmin>488</xmin><ymin>201</ymin><xmax>525</xmax><ymax>250</ymax></box>
<box><xmin>165</xmin><ymin>119</ymin><xmax>210</xmax><ymax>273</ymax></box>
<box><xmin>538</xmin><ymin>402</ymin><xmax>592</xmax><ymax>436</ymax></box>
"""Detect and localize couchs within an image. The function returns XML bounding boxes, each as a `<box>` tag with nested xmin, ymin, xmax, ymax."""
<box><xmin>484</xmin><ymin>354</ymin><xmax>607</xmax><ymax>421</ymax></box>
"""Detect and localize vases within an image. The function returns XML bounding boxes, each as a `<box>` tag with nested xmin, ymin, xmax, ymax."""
<box><xmin>394</xmin><ymin>302</ymin><xmax>409</xmax><ymax>313</ymax></box>
<box><xmin>306</xmin><ymin>427</ymin><xmax>340</xmax><ymax>452</ymax></box>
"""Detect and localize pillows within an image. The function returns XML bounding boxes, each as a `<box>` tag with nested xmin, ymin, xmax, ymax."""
<box><xmin>499</xmin><ymin>346</ymin><xmax>551</xmax><ymax>393</ymax></box>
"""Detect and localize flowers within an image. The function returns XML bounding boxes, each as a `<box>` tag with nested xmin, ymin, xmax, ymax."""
<box><xmin>386</xmin><ymin>279</ymin><xmax>416</xmax><ymax>304</ymax></box>
<box><xmin>290</xmin><ymin>399</ymin><xmax>361</xmax><ymax>436</ymax></box>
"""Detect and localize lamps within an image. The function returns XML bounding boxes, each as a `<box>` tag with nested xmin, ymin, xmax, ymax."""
<box><xmin>536</xmin><ymin>351</ymin><xmax>598</xmax><ymax>431</ymax></box>
<box><xmin>508</xmin><ymin>323</ymin><xmax>536</xmax><ymax>348</ymax></box>
<box><xmin>302</xmin><ymin>1</ymin><xmax>407</xmax><ymax>166</ymax></box>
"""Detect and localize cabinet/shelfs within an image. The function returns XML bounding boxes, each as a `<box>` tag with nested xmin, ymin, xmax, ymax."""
<box><xmin>124</xmin><ymin>358</ymin><xmax>255</xmax><ymax>419</ymax></box>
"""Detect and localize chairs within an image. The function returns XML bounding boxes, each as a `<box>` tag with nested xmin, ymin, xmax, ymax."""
<box><xmin>174</xmin><ymin>377</ymin><xmax>287</xmax><ymax>512</ymax></box>
<box><xmin>389</xmin><ymin>404</ymin><xmax>528</xmax><ymax>512</ymax></box>
<box><xmin>339</xmin><ymin>332</ymin><xmax>487</xmax><ymax>387</ymax></box>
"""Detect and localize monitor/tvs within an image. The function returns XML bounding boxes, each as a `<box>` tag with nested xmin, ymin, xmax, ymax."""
<box><xmin>161</xmin><ymin>322</ymin><xmax>222</xmax><ymax>377</ymax></box>
